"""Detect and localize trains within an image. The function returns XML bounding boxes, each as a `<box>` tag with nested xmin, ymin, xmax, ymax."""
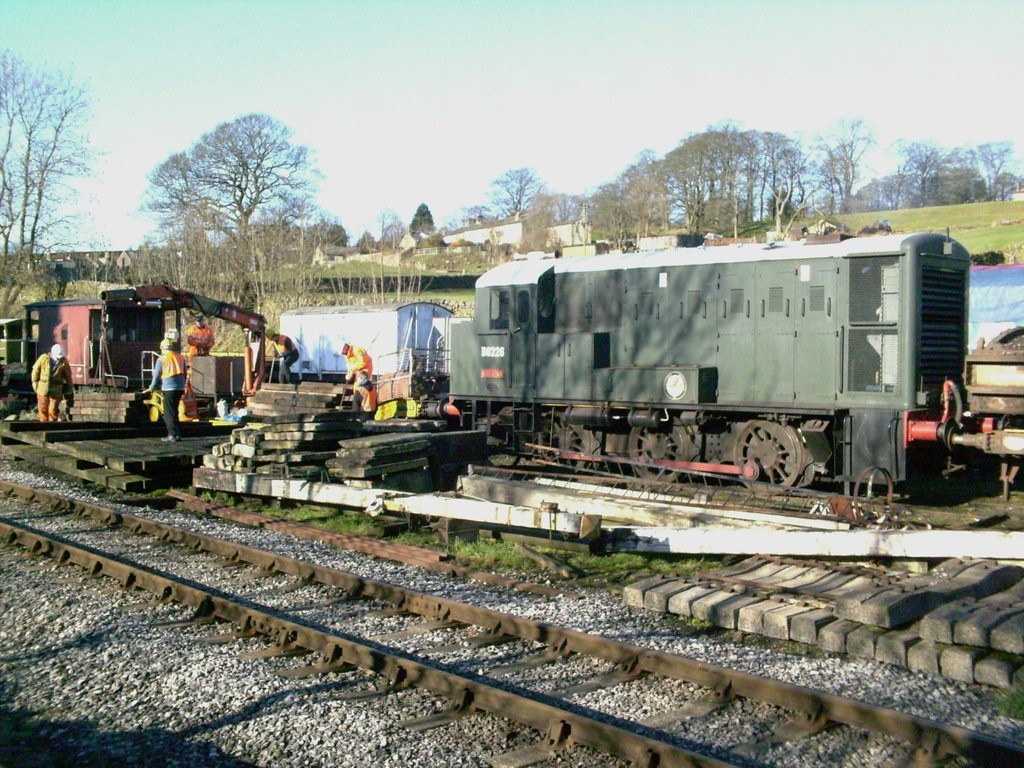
<box><xmin>280</xmin><ymin>300</ymin><xmax>454</xmax><ymax>382</ymax></box>
<box><xmin>0</xmin><ymin>299</ymin><xmax>166</xmax><ymax>389</ymax></box>
<box><xmin>445</xmin><ymin>226</ymin><xmax>1024</xmax><ymax>499</ymax></box>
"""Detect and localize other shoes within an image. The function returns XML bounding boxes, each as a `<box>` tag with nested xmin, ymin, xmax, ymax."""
<box><xmin>161</xmin><ymin>435</ymin><xmax>182</xmax><ymax>443</ymax></box>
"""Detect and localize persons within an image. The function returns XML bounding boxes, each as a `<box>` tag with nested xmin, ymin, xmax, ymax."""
<box><xmin>30</xmin><ymin>343</ymin><xmax>75</xmax><ymax>422</ymax></box>
<box><xmin>265</xmin><ymin>328</ymin><xmax>300</xmax><ymax>384</ymax></box>
<box><xmin>355</xmin><ymin>371</ymin><xmax>376</xmax><ymax>438</ymax></box>
<box><xmin>149</xmin><ymin>338</ymin><xmax>188</xmax><ymax>444</ymax></box>
<box><xmin>337</xmin><ymin>342</ymin><xmax>376</xmax><ymax>423</ymax></box>
<box><xmin>186</xmin><ymin>316</ymin><xmax>216</xmax><ymax>382</ymax></box>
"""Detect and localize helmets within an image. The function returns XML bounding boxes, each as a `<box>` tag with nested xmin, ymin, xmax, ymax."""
<box><xmin>160</xmin><ymin>339</ymin><xmax>173</xmax><ymax>349</ymax></box>
<box><xmin>337</xmin><ymin>341</ymin><xmax>346</xmax><ymax>355</ymax></box>
<box><xmin>265</xmin><ymin>329</ymin><xmax>275</xmax><ymax>340</ymax></box>
<box><xmin>196</xmin><ymin>316</ymin><xmax>203</xmax><ymax>321</ymax></box>
<box><xmin>50</xmin><ymin>344</ymin><xmax>64</xmax><ymax>359</ymax></box>
